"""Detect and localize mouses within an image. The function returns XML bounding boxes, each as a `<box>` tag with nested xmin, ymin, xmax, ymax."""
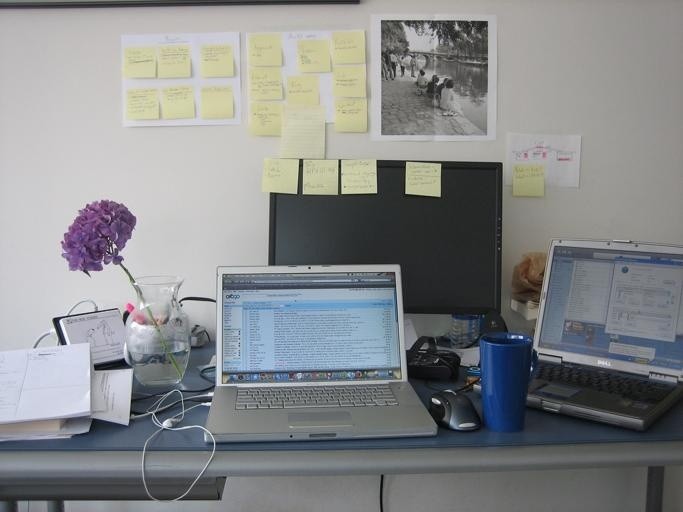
<box><xmin>428</xmin><ymin>390</ymin><xmax>480</xmax><ymax>432</ymax></box>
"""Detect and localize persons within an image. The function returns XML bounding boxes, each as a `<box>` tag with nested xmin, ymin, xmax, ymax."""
<box><xmin>381</xmin><ymin>50</ymin><xmax>416</xmax><ymax>80</ymax></box>
<box><xmin>417</xmin><ymin>69</ymin><xmax>463</xmax><ymax>116</ymax></box>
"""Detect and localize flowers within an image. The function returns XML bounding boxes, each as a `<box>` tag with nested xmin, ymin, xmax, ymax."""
<box><xmin>60</xmin><ymin>199</ymin><xmax>185</xmax><ymax>379</ymax></box>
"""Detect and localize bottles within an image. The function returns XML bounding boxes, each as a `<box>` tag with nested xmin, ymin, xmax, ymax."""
<box><xmin>449</xmin><ymin>313</ymin><xmax>484</xmax><ymax>349</ymax></box>
<box><xmin>478</xmin><ymin>331</ymin><xmax>534</xmax><ymax>432</ymax></box>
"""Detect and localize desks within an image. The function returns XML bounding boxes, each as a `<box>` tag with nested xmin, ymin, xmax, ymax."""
<box><xmin>1</xmin><ymin>340</ymin><xmax>683</xmax><ymax>510</ymax></box>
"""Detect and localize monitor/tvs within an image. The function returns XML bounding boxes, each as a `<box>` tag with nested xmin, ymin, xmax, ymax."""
<box><xmin>269</xmin><ymin>159</ymin><xmax>502</xmax><ymax>314</ymax></box>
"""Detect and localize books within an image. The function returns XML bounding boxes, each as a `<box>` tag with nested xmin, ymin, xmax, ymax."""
<box><xmin>0</xmin><ymin>309</ymin><xmax>134</xmax><ymax>441</ymax></box>
<box><xmin>511</xmin><ymin>299</ymin><xmax>539</xmax><ymax>321</ymax></box>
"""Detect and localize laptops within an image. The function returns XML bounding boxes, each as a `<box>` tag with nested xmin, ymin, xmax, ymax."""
<box><xmin>203</xmin><ymin>263</ymin><xmax>439</xmax><ymax>444</ymax></box>
<box><xmin>526</xmin><ymin>239</ymin><xmax>683</xmax><ymax>433</ymax></box>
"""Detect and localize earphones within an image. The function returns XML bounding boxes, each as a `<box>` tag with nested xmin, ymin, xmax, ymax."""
<box><xmin>162</xmin><ymin>418</ymin><xmax>181</xmax><ymax>429</ymax></box>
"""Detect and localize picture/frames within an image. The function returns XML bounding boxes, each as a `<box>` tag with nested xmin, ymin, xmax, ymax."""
<box><xmin>369</xmin><ymin>12</ymin><xmax>496</xmax><ymax>142</ymax></box>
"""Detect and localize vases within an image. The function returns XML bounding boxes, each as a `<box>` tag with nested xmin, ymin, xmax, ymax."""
<box><xmin>124</xmin><ymin>275</ymin><xmax>190</xmax><ymax>386</ymax></box>
